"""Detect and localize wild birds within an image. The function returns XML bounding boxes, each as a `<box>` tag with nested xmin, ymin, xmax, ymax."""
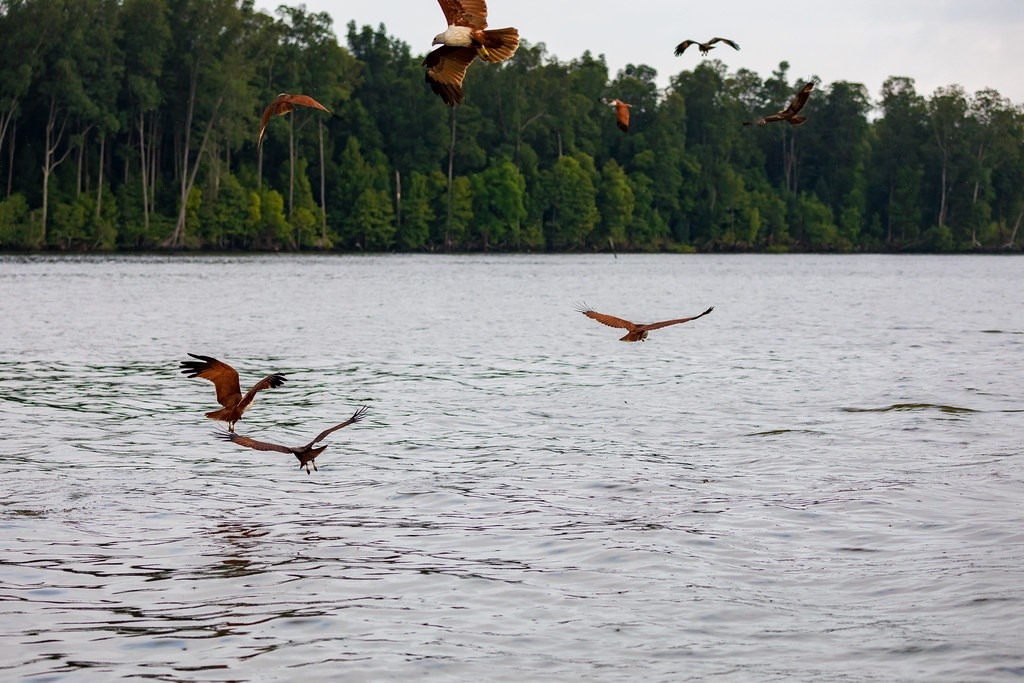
<box><xmin>422</xmin><ymin>0</ymin><xmax>519</xmax><ymax>108</ymax></box>
<box><xmin>598</xmin><ymin>97</ymin><xmax>633</xmax><ymax>134</ymax></box>
<box><xmin>743</xmin><ymin>75</ymin><xmax>818</xmax><ymax>126</ymax></box>
<box><xmin>675</xmin><ymin>37</ymin><xmax>740</xmax><ymax>57</ymax></box>
<box><xmin>258</xmin><ymin>93</ymin><xmax>333</xmax><ymax>151</ymax></box>
<box><xmin>182</xmin><ymin>351</ymin><xmax>287</xmax><ymax>432</ymax></box>
<box><xmin>213</xmin><ymin>404</ymin><xmax>370</xmax><ymax>475</ymax></box>
<box><xmin>573</xmin><ymin>299</ymin><xmax>714</xmax><ymax>342</ymax></box>
